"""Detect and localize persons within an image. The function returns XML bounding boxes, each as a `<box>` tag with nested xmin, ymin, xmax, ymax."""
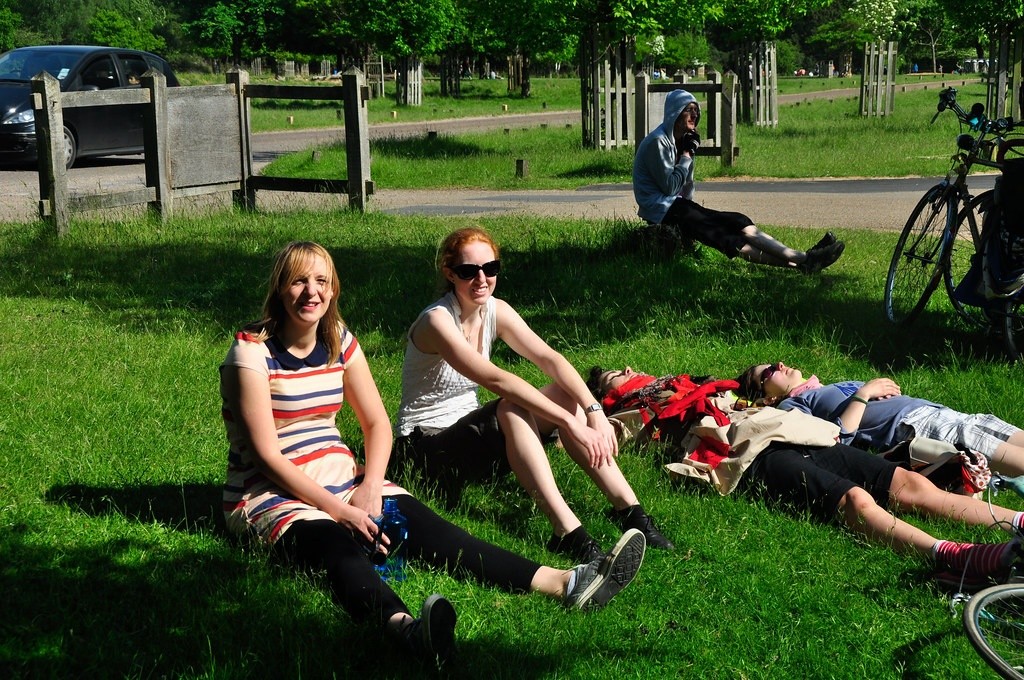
<box><xmin>557</xmin><ymin>362</ymin><xmax>1024</xmax><ymax>582</ymax></box>
<box><xmin>738</xmin><ymin>360</ymin><xmax>1024</xmax><ymax>477</ymax></box>
<box><xmin>396</xmin><ymin>229</ymin><xmax>675</xmax><ymax>563</ymax></box>
<box><xmin>127</xmin><ymin>71</ymin><xmax>141</xmax><ymax>85</ymax></box>
<box><xmin>218</xmin><ymin>239</ymin><xmax>646</xmax><ymax>677</ymax></box>
<box><xmin>631</xmin><ymin>86</ymin><xmax>846</xmax><ymax>274</ymax></box>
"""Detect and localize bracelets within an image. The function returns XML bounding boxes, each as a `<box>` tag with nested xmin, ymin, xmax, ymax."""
<box><xmin>851</xmin><ymin>395</ymin><xmax>868</xmax><ymax>405</ymax></box>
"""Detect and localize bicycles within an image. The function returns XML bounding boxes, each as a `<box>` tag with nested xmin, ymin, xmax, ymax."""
<box><xmin>950</xmin><ymin>471</ymin><xmax>1024</xmax><ymax>680</ymax></box>
<box><xmin>885</xmin><ymin>85</ymin><xmax>1024</xmax><ymax>363</ymax></box>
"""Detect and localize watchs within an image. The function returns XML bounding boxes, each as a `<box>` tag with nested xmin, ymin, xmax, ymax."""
<box><xmin>584</xmin><ymin>403</ymin><xmax>603</xmax><ymax>417</ymax></box>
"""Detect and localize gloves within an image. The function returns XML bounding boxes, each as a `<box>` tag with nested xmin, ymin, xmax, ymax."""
<box><xmin>682</xmin><ymin>128</ymin><xmax>701</xmax><ymax>156</ymax></box>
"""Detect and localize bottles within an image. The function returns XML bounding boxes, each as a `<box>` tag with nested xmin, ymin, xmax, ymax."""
<box><xmin>369</xmin><ymin>498</ymin><xmax>408</xmax><ymax>584</ymax></box>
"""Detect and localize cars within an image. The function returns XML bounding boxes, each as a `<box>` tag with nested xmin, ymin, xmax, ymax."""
<box><xmin>0</xmin><ymin>46</ymin><xmax>180</xmax><ymax>170</ymax></box>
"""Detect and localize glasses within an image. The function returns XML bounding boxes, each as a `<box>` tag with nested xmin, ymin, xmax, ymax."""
<box><xmin>372</xmin><ymin>519</ymin><xmax>388</xmax><ymax>566</ymax></box>
<box><xmin>760</xmin><ymin>364</ymin><xmax>775</xmax><ymax>398</ymax></box>
<box><xmin>447</xmin><ymin>259</ymin><xmax>502</xmax><ymax>282</ymax></box>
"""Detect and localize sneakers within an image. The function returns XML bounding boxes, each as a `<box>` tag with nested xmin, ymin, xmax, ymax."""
<box><xmin>806</xmin><ymin>232</ymin><xmax>836</xmax><ymax>254</ymax></box>
<box><xmin>609</xmin><ymin>503</ymin><xmax>675</xmax><ymax>552</ymax></box>
<box><xmin>403</xmin><ymin>595</ymin><xmax>457</xmax><ymax>680</ymax></box>
<box><xmin>547</xmin><ymin>523</ymin><xmax>603</xmax><ymax>563</ymax></box>
<box><xmin>798</xmin><ymin>240</ymin><xmax>844</xmax><ymax>274</ymax></box>
<box><xmin>566</xmin><ymin>528</ymin><xmax>647</xmax><ymax>615</ymax></box>
